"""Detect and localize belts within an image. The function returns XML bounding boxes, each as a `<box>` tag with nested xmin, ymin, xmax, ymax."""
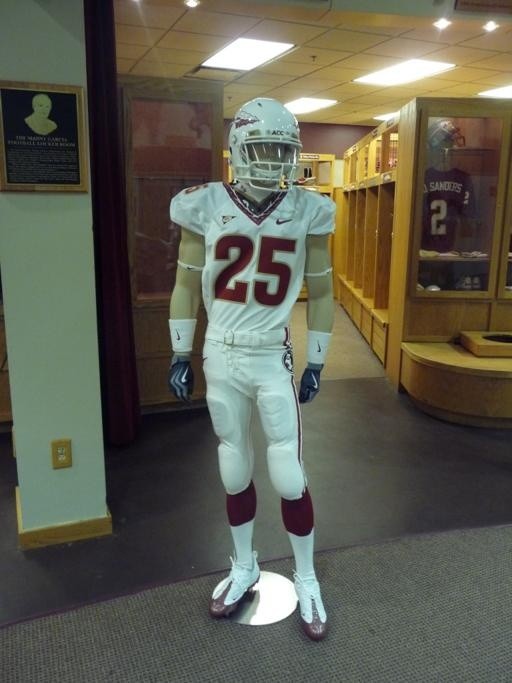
<box><xmin>206</xmin><ymin>325</ymin><xmax>290</xmax><ymax>346</ymax></box>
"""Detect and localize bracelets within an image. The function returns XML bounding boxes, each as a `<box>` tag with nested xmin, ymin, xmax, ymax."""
<box><xmin>302</xmin><ymin>328</ymin><xmax>335</xmax><ymax>368</ymax></box>
<box><xmin>165</xmin><ymin>317</ymin><xmax>200</xmax><ymax>356</ymax></box>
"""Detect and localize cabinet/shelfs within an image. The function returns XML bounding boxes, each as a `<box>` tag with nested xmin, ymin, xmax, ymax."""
<box><xmin>333</xmin><ymin>94</ymin><xmax>512</xmax><ymax>430</ymax></box>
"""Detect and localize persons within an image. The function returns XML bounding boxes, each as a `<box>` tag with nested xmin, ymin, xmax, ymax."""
<box><xmin>167</xmin><ymin>98</ymin><xmax>337</xmax><ymax>641</ymax></box>
<box><xmin>10</xmin><ymin>93</ymin><xmax>67</xmax><ymax>149</ymax></box>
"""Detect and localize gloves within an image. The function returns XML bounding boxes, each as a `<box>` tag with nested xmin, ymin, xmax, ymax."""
<box><xmin>166</xmin><ymin>354</ymin><xmax>193</xmax><ymax>402</ymax></box>
<box><xmin>299</xmin><ymin>364</ymin><xmax>322</xmax><ymax>403</ymax></box>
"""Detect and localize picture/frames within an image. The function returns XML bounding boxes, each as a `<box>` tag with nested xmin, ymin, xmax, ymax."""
<box><xmin>0</xmin><ymin>80</ymin><xmax>89</xmax><ymax>195</ymax></box>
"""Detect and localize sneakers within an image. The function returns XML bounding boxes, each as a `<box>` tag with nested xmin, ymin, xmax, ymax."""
<box><xmin>295</xmin><ymin>572</ymin><xmax>328</xmax><ymax>640</ymax></box>
<box><xmin>210</xmin><ymin>551</ymin><xmax>260</xmax><ymax>618</ymax></box>
<box><xmin>454</xmin><ymin>274</ymin><xmax>480</xmax><ymax>290</ymax></box>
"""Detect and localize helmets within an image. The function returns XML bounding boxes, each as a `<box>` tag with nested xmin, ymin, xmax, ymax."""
<box><xmin>428</xmin><ymin>119</ymin><xmax>466</xmax><ymax>147</ymax></box>
<box><xmin>228</xmin><ymin>98</ymin><xmax>299</xmax><ymax>193</ymax></box>
